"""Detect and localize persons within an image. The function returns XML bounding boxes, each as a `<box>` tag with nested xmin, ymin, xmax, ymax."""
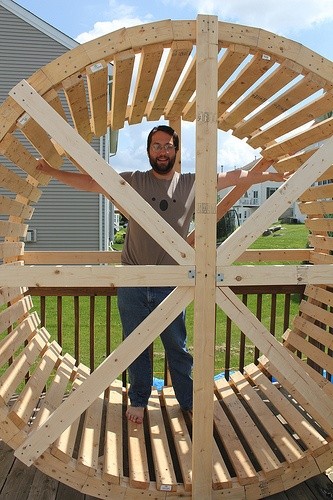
<box><xmin>35</xmin><ymin>125</ymin><xmax>295</xmax><ymax>425</ymax></box>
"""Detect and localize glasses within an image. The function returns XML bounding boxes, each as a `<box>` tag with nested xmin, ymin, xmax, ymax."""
<box><xmin>150</xmin><ymin>143</ymin><xmax>176</xmax><ymax>152</ymax></box>
